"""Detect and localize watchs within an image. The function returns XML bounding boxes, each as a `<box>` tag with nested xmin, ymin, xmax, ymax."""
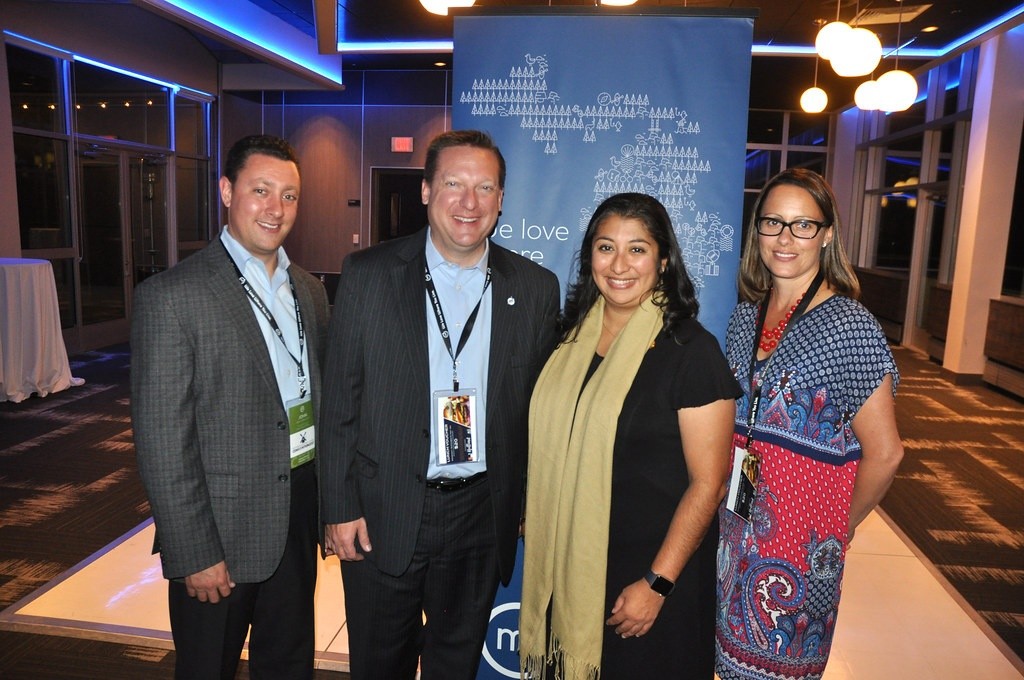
<box><xmin>644</xmin><ymin>570</ymin><xmax>674</xmax><ymax>598</ymax></box>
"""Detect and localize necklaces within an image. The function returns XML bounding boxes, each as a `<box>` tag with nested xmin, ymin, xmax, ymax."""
<box><xmin>759</xmin><ymin>291</ymin><xmax>805</xmax><ymax>351</ymax></box>
<box><xmin>603</xmin><ymin>323</ymin><xmax>616</xmax><ymax>337</ymax></box>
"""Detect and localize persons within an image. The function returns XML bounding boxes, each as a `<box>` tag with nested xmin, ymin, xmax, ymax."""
<box><xmin>517</xmin><ymin>193</ymin><xmax>744</xmax><ymax>680</ymax></box>
<box><xmin>715</xmin><ymin>169</ymin><xmax>904</xmax><ymax>680</ymax></box>
<box><xmin>316</xmin><ymin>131</ymin><xmax>560</xmax><ymax>680</ymax></box>
<box><xmin>129</xmin><ymin>134</ymin><xmax>329</xmax><ymax>680</ymax></box>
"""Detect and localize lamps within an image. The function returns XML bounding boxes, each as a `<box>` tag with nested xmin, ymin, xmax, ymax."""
<box><xmin>800</xmin><ymin>0</ymin><xmax>918</xmax><ymax>114</ymax></box>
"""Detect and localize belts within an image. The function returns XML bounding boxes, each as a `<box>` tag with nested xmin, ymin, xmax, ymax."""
<box><xmin>425</xmin><ymin>471</ymin><xmax>488</xmax><ymax>492</ymax></box>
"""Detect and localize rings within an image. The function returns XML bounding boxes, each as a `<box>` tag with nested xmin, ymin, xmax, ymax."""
<box><xmin>324</xmin><ymin>548</ymin><xmax>332</xmax><ymax>553</ymax></box>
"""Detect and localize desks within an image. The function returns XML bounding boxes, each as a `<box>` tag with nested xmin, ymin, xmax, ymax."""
<box><xmin>0</xmin><ymin>257</ymin><xmax>85</xmax><ymax>404</ymax></box>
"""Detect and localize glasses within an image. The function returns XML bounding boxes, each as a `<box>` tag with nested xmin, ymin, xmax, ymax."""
<box><xmin>755</xmin><ymin>216</ymin><xmax>827</xmax><ymax>240</ymax></box>
